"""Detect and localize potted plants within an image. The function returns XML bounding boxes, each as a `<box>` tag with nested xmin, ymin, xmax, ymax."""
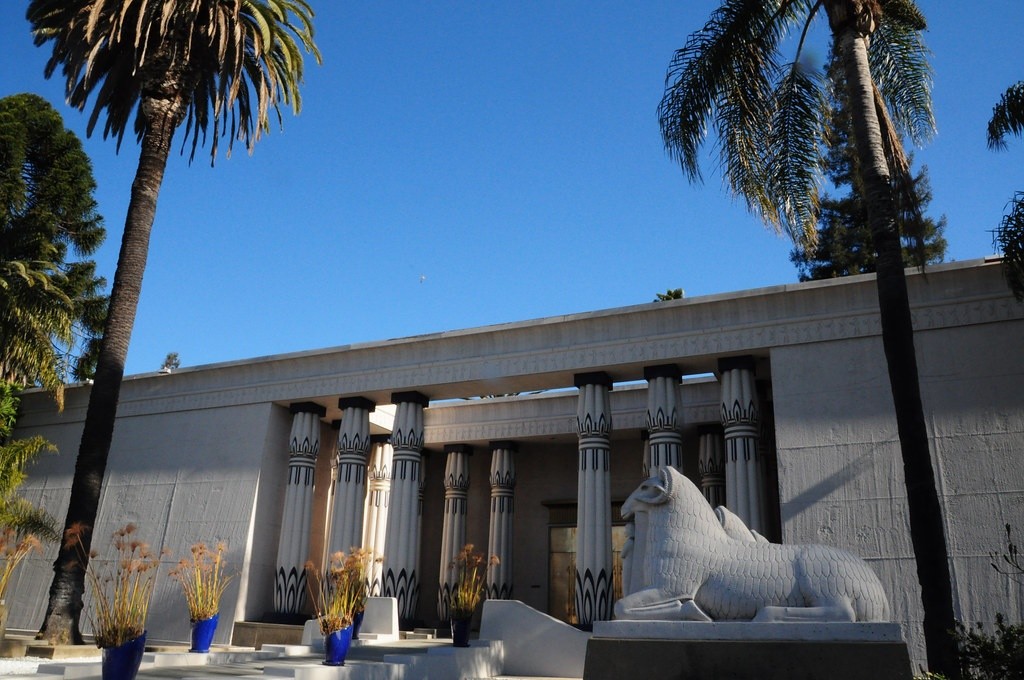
<box><xmin>66</xmin><ymin>523</ymin><xmax>173</xmax><ymax>680</ymax></box>
<box><xmin>439</xmin><ymin>544</ymin><xmax>500</xmax><ymax>647</ymax></box>
<box><xmin>176</xmin><ymin>542</ymin><xmax>249</xmax><ymax>655</ymax></box>
<box><xmin>301</xmin><ymin>545</ymin><xmax>385</xmax><ymax>666</ymax></box>
